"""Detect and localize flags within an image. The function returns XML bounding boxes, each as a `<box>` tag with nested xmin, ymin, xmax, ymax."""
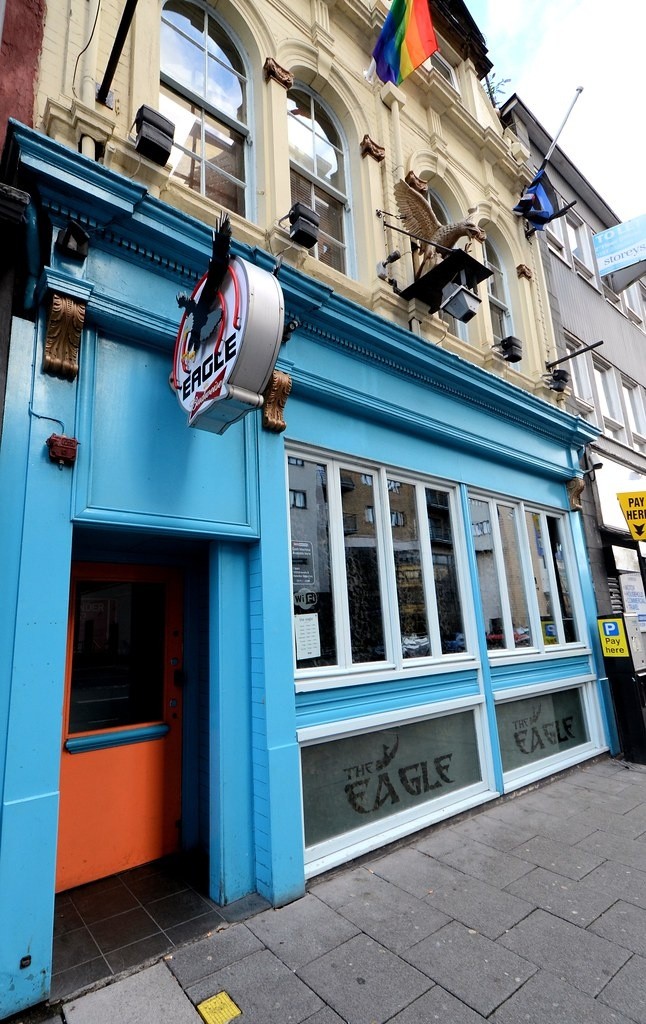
<box><xmin>372</xmin><ymin>0</ymin><xmax>438</xmax><ymax>87</ymax></box>
<box><xmin>513</xmin><ymin>170</ymin><xmax>568</xmax><ymax>231</ymax></box>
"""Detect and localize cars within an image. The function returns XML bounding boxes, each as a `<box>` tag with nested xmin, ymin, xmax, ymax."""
<box><xmin>374</xmin><ymin>628</ymin><xmax>531</xmax><ymax>660</ymax></box>
